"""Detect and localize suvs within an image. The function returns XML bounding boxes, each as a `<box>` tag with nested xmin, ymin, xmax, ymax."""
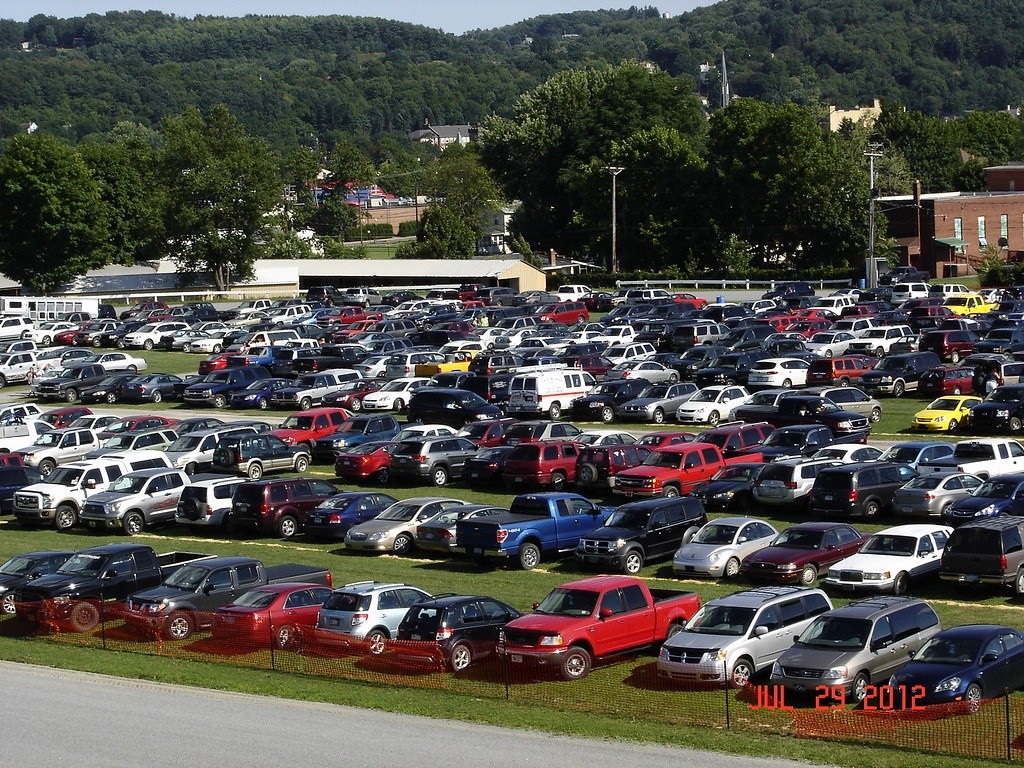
<box><xmin>938</xmin><ymin>513</ymin><xmax>1024</xmax><ymax>601</ymax></box>
<box><xmin>230</xmin><ymin>476</ymin><xmax>347</xmax><ymax>539</ymax></box>
<box><xmin>657</xmin><ymin>585</ymin><xmax>836</xmax><ymax>691</ymax></box>
<box><xmin>572</xmin><ymin>496</ymin><xmax>709</xmax><ymax>576</ymax></box>
<box><xmin>174</xmin><ymin>476</ymin><xmax>261</xmax><ymax>534</ymax></box>
<box><xmin>314</xmin><ymin>580</ymin><xmax>477</xmax><ymax>658</ymax></box>
<box><xmin>768</xmin><ymin>595</ymin><xmax>945</xmax><ymax>704</ymax></box>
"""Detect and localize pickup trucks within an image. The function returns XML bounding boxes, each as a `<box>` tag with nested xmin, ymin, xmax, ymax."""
<box><xmin>78</xmin><ymin>467</ymin><xmax>237</xmax><ymax>536</ymax></box>
<box><xmin>123</xmin><ymin>556</ymin><xmax>333</xmax><ymax>641</ymax></box>
<box><xmin>456</xmin><ymin>491</ymin><xmax>625</xmax><ymax>571</ymax></box>
<box><xmin>879</xmin><ymin>266</ymin><xmax>932</xmax><ymax>286</ymax></box>
<box><xmin>12</xmin><ymin>543</ymin><xmax>218</xmax><ymax>634</ymax></box>
<box><xmin>494</xmin><ymin>576</ymin><xmax>701</xmax><ymax>681</ymax></box>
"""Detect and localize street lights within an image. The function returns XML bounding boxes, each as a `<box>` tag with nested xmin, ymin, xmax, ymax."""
<box><xmin>601</xmin><ymin>166</ymin><xmax>625</xmax><ymax>274</ymax></box>
<box><xmin>861</xmin><ymin>142</ymin><xmax>884</xmax><ymax>289</ymax></box>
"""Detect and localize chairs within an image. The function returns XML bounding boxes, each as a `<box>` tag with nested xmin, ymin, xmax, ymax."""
<box><xmin>419</xmin><ymin>605</ymin><xmax>480</xmax><ymax>626</ymax></box>
<box><xmin>244</xmin><ymin>440</ymin><xmax>265</xmax><ymax>450</ymax></box>
<box><xmin>333</xmin><ymin>594</ymin><xmax>394</xmax><ymax>611</ymax></box>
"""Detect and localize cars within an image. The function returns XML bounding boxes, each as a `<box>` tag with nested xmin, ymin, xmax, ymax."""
<box><xmin>344</xmin><ymin>497</ymin><xmax>474</xmax><ymax>560</ymax></box>
<box><xmin>0</xmin><ymin>282</ymin><xmax>1024</xmax><ymax>534</ymax></box>
<box><xmin>415</xmin><ymin>504</ymin><xmax>510</xmax><ymax>563</ymax></box>
<box><xmin>824</xmin><ymin>523</ymin><xmax>955</xmax><ymax>597</ymax></box>
<box><xmin>395</xmin><ymin>592</ymin><xmax>527</xmax><ymax>675</ymax></box>
<box><xmin>1</xmin><ymin>550</ymin><xmax>82</xmax><ymax>615</ymax></box>
<box><xmin>884</xmin><ymin>622</ymin><xmax>1024</xmax><ymax>715</ymax></box>
<box><xmin>739</xmin><ymin>522</ymin><xmax>876</xmax><ymax>588</ymax></box>
<box><xmin>672</xmin><ymin>517</ymin><xmax>787</xmax><ymax>581</ymax></box>
<box><xmin>211</xmin><ymin>582</ymin><xmax>357</xmax><ymax>651</ymax></box>
<box><xmin>304</xmin><ymin>491</ymin><xmax>400</xmax><ymax>539</ymax></box>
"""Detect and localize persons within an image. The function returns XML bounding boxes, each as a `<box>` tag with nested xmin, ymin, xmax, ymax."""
<box><xmin>24</xmin><ymin>363</ymin><xmax>52</xmax><ymax>397</ymax></box>
<box><xmin>472</xmin><ymin>313</ymin><xmax>489</xmax><ymax>327</ymax></box>
